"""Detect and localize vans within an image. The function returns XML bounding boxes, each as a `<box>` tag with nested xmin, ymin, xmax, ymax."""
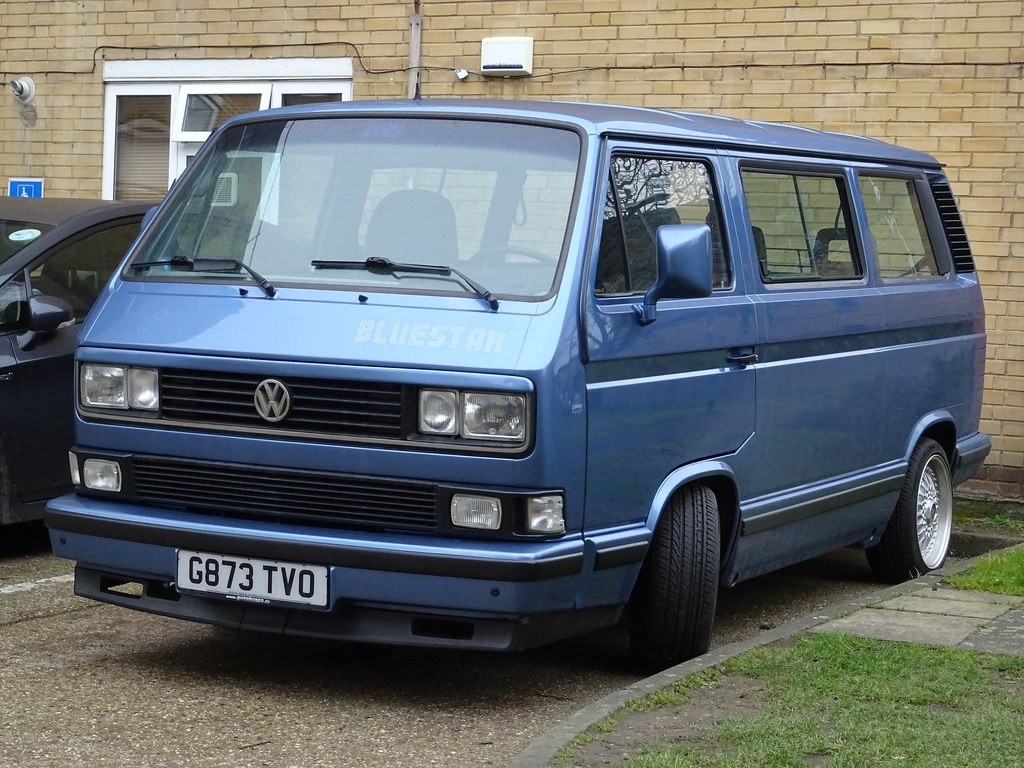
<box><xmin>45</xmin><ymin>99</ymin><xmax>992</xmax><ymax>676</ymax></box>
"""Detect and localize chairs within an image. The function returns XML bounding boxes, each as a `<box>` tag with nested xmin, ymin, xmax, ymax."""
<box><xmin>813</xmin><ymin>228</ymin><xmax>880</xmax><ymax>278</ymax></box>
<box><xmin>365</xmin><ymin>189</ymin><xmax>457</xmax><ymax>266</ymax></box>
<box><xmin>38</xmin><ymin>233</ymin><xmax>104</xmax><ymax>301</ymax></box>
<box><xmin>712</xmin><ymin>227</ymin><xmax>769</xmax><ymax>287</ymax></box>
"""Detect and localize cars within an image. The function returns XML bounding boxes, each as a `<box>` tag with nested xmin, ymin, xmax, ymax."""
<box><xmin>0</xmin><ymin>196</ymin><xmax>312</xmax><ymax>528</ymax></box>
